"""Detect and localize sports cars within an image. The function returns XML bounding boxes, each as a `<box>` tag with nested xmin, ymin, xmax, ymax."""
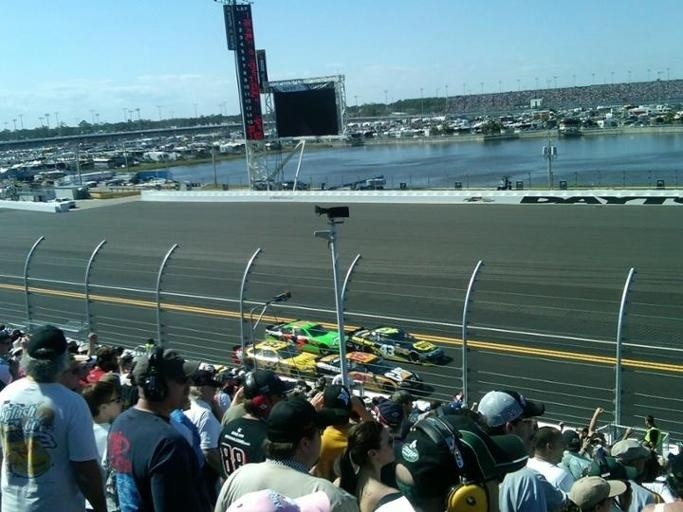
<box><xmin>346</xmin><ymin>326</ymin><xmax>445</xmax><ymax>364</ymax></box>
<box><xmin>230</xmin><ymin>341</ymin><xmax>320</xmax><ymax>378</ymax></box>
<box><xmin>264</xmin><ymin>320</ymin><xmax>348</xmax><ymax>355</ymax></box>
<box><xmin>315</xmin><ymin>351</ymin><xmax>423</xmax><ymax>393</ymax></box>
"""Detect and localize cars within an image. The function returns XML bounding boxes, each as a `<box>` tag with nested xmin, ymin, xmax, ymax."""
<box><xmin>348</xmin><ymin>104</ymin><xmax>683</xmax><ymax>141</ymax></box>
<box><xmin>262</xmin><ymin>124</ymin><xmax>281</xmax><ymax>150</ymax></box>
<box><xmin>1</xmin><ymin>132</ymin><xmax>246</xmax><ymax>187</ymax></box>
<box><xmin>252</xmin><ymin>177</ymin><xmax>275</xmax><ymax>189</ymax></box>
<box><xmin>53</xmin><ymin>196</ymin><xmax>76</xmax><ymax>208</ymax></box>
<box><xmin>281</xmin><ymin>180</ymin><xmax>309</xmax><ymax>191</ymax></box>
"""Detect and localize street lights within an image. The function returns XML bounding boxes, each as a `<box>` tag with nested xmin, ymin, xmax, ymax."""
<box><xmin>444</xmin><ymin>85</ymin><xmax>448</xmax><ymax>114</ymax></box>
<box><xmin>4</xmin><ymin>100</ymin><xmax>225</xmax><ymax>140</ymax></box>
<box><xmin>419</xmin><ymin>87</ymin><xmax>424</xmax><ymax>116</ymax></box>
<box><xmin>384</xmin><ymin>90</ymin><xmax>389</xmax><ymax>115</ymax></box>
<box><xmin>461</xmin><ymin>66</ymin><xmax>670</xmax><ymax>109</ymax></box>
<box><xmin>354</xmin><ymin>95</ymin><xmax>358</xmax><ymax>119</ymax></box>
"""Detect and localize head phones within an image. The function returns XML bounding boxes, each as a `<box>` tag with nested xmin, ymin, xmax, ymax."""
<box><xmin>412</xmin><ymin>413</ymin><xmax>490</xmax><ymax>512</ymax></box>
<box><xmin>245</xmin><ymin>372</ymin><xmax>273</xmax><ymax>413</ymax></box>
<box><xmin>144</xmin><ymin>346</ymin><xmax>168</xmax><ymax>402</ymax></box>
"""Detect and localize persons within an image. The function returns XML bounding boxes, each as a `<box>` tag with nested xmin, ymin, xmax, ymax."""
<box><xmin>0</xmin><ymin>323</ymin><xmax>683</xmax><ymax>512</ymax></box>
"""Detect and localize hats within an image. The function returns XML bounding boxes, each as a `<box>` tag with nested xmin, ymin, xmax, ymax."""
<box><xmin>568</xmin><ymin>476</ymin><xmax>627</xmax><ymax>510</ymax></box>
<box><xmin>131</xmin><ymin>347</ymin><xmax>219</xmax><ymax>386</ymax></box>
<box><xmin>611</xmin><ymin>440</ymin><xmax>650</xmax><ymax>464</ymax></box>
<box><xmin>243</xmin><ymin>370</ymin><xmax>350</xmax><ymax>442</ymax></box>
<box><xmin>27</xmin><ymin>325</ymin><xmax>67</xmax><ymax>359</ymax></box>
<box><xmin>391</xmin><ymin>391</ymin><xmax>544</xmax><ymax>498</ymax></box>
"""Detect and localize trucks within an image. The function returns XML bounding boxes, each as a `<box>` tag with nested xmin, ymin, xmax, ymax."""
<box><xmin>352</xmin><ymin>177</ymin><xmax>385</xmax><ymax>189</ymax></box>
